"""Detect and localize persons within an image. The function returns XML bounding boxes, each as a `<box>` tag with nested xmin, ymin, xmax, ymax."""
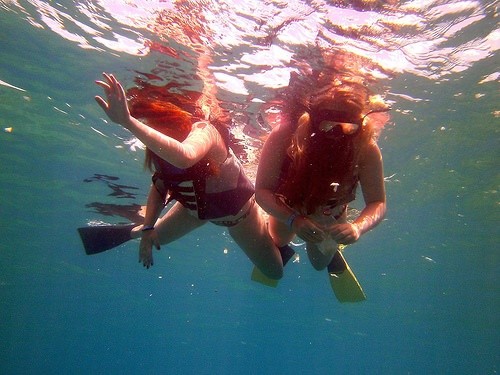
<box><xmin>76</xmin><ymin>72</ymin><xmax>284</xmax><ymax>280</ymax></box>
<box><xmin>255</xmin><ymin>79</ymin><xmax>386</xmax><ymax>249</ymax></box>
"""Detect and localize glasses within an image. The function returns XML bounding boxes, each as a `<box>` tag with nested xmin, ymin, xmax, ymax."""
<box><xmin>318</xmin><ymin>120</ymin><xmax>359</xmax><ymax>135</ymax></box>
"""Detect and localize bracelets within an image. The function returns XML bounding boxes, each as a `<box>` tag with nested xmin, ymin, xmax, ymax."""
<box><xmin>140</xmin><ymin>224</ymin><xmax>155</xmax><ymax>232</ymax></box>
<box><xmin>286</xmin><ymin>211</ymin><xmax>303</xmax><ymax>231</ymax></box>
